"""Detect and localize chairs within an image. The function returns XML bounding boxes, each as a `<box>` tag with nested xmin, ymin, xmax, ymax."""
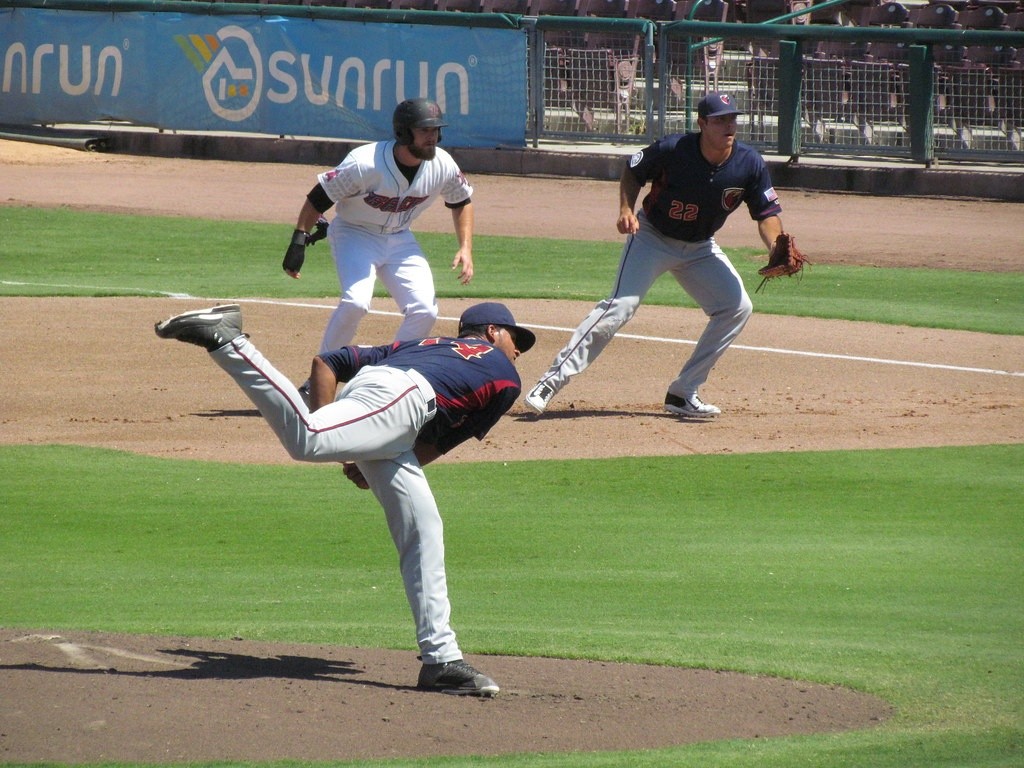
<box><xmin>300</xmin><ymin>0</ymin><xmax>1024</xmax><ymax>152</ymax></box>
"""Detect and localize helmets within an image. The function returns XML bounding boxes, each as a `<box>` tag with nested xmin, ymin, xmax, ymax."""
<box><xmin>392</xmin><ymin>98</ymin><xmax>448</xmax><ymax>145</ymax></box>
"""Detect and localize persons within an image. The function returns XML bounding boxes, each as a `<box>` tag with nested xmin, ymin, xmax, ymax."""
<box><xmin>524</xmin><ymin>92</ymin><xmax>805</xmax><ymax>418</ymax></box>
<box><xmin>283</xmin><ymin>99</ymin><xmax>476</xmax><ymax>394</ymax></box>
<box><xmin>153</xmin><ymin>303</ymin><xmax>536</xmax><ymax>698</ymax></box>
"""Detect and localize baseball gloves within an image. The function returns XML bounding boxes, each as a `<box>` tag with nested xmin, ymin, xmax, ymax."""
<box><xmin>754</xmin><ymin>231</ymin><xmax>814</xmax><ymax>296</ymax></box>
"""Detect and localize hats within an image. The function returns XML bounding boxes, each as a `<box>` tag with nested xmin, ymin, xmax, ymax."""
<box><xmin>458</xmin><ymin>302</ymin><xmax>535</xmax><ymax>354</ymax></box>
<box><xmin>698</xmin><ymin>92</ymin><xmax>745</xmax><ymax>119</ymax></box>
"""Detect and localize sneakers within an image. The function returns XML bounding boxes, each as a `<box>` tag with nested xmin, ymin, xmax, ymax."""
<box><xmin>525</xmin><ymin>381</ymin><xmax>556</xmax><ymax>413</ymax></box>
<box><xmin>416</xmin><ymin>660</ymin><xmax>499</xmax><ymax>697</ymax></box>
<box><xmin>663</xmin><ymin>390</ymin><xmax>721</xmax><ymax>418</ymax></box>
<box><xmin>154</xmin><ymin>303</ymin><xmax>244</xmax><ymax>350</ymax></box>
